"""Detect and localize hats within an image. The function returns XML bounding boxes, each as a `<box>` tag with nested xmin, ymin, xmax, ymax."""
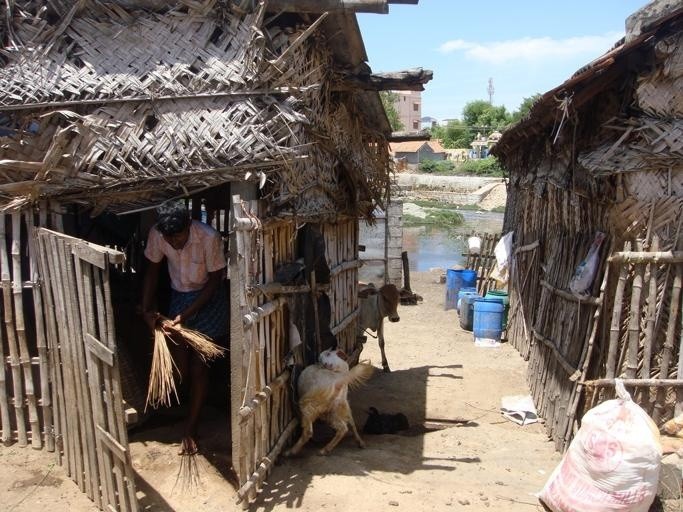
<box><xmin>153</xmin><ymin>196</ymin><xmax>189</xmax><ymax>227</ymax></box>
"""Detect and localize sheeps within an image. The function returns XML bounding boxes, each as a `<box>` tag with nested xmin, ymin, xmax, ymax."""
<box><xmin>281</xmin><ymin>348</ymin><xmax>375</xmax><ymax>458</ymax></box>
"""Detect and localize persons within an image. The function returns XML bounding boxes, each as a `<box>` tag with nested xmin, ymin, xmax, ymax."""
<box><xmin>139</xmin><ymin>202</ymin><xmax>226</xmax><ymax>455</ymax></box>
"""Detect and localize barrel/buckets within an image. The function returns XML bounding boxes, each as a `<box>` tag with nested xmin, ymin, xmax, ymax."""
<box><xmin>443</xmin><ymin>269</ymin><xmax>477</xmax><ymax>311</ymax></box>
<box><xmin>485</xmin><ymin>291</ymin><xmax>511</xmax><ymax>331</ymax></box>
<box><xmin>471</xmin><ymin>298</ymin><xmax>503</xmax><ymax>342</ymax></box>
<box><xmin>456</xmin><ymin>286</ymin><xmax>478</xmax><ymax>314</ymax></box>
<box><xmin>467</xmin><ymin>236</ymin><xmax>481</xmax><ymax>253</ymax></box>
<box><xmin>459</xmin><ymin>292</ymin><xmax>483</xmax><ymax>330</ymax></box>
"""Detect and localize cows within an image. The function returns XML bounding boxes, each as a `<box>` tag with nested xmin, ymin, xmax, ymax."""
<box><xmin>358</xmin><ymin>283</ymin><xmax>400</xmax><ymax>373</ymax></box>
